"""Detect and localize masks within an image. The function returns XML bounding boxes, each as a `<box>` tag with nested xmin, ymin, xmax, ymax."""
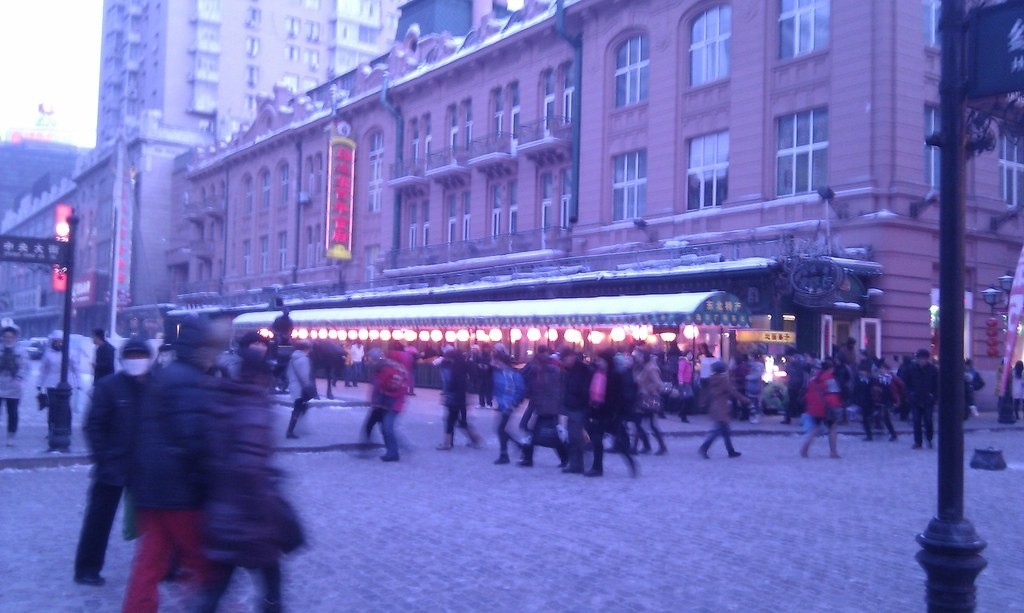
<box><xmin>119</xmin><ymin>357</ymin><xmax>151</xmax><ymax>376</ymax></box>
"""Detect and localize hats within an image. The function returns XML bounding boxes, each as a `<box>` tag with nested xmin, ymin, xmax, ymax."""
<box><xmin>711</xmin><ymin>361</ymin><xmax>729</xmax><ymax>374</ymax></box>
<box><xmin>597</xmin><ymin>347</ymin><xmax>617</xmax><ymax>363</ymax></box>
<box><xmin>122</xmin><ymin>337</ymin><xmax>151</xmax><ymax>354</ymax></box>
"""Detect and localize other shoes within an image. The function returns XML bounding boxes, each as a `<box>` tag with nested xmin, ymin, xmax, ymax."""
<box><xmin>800</xmin><ymin>447</ymin><xmax>808</xmax><ymax>458</ymax></box>
<box><xmin>912</xmin><ymin>443</ymin><xmax>921</xmax><ymax>449</ymax></box>
<box><xmin>830</xmin><ymin>452</ymin><xmax>841</xmax><ymax>458</ymax></box>
<box><xmin>376</xmin><ymin>437</ymin><xmax>742</xmax><ymax>476</ymax></box>
<box><xmin>74</xmin><ymin>571</ymin><xmax>106</xmax><ymax>586</ymax></box>
<box><xmin>6</xmin><ymin>432</ymin><xmax>15</xmax><ymax>446</ymax></box>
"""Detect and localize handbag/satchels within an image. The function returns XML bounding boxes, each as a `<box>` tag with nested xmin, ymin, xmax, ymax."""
<box><xmin>639</xmin><ymin>395</ymin><xmax>664</xmax><ymax>411</ymax></box>
<box><xmin>800</xmin><ymin>413</ymin><xmax>829</xmax><ymax>437</ymax></box>
<box><xmin>972</xmin><ymin>373</ymin><xmax>985</xmax><ymax>391</ymax></box>
<box><xmin>302</xmin><ymin>386</ymin><xmax>317</xmax><ymax>402</ymax></box>
<box><xmin>35</xmin><ymin>392</ymin><xmax>49</xmax><ymax>412</ymax></box>
<box><xmin>825</xmin><ymin>407</ymin><xmax>843</xmax><ymax>423</ymax></box>
<box><xmin>73</xmin><ymin>390</ymin><xmax>86</xmax><ymax>413</ymax></box>
<box><xmin>534</xmin><ymin>425</ymin><xmax>563</xmax><ymax>448</ymax></box>
<box><xmin>371</xmin><ymin>390</ymin><xmax>397</xmax><ymax>411</ymax></box>
<box><xmin>122</xmin><ymin>491</ymin><xmax>139</xmax><ymax>540</ymax></box>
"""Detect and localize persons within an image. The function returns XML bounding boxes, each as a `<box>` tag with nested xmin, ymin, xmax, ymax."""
<box><xmin>73</xmin><ymin>321</ymin><xmax>306</xmax><ymax>613</ymax></box>
<box><xmin>697</xmin><ymin>343</ymin><xmax>765</xmax><ymax>458</ymax></box>
<box><xmin>270</xmin><ymin>307</ymin><xmax>436</xmax><ymax>462</ymax></box>
<box><xmin>36</xmin><ymin>331</ymin><xmax>80</xmax><ymax>438</ymax></box>
<box><xmin>435</xmin><ymin>340</ymin><xmax>695</xmax><ymax>477</ymax></box>
<box><xmin>781</xmin><ymin>338</ymin><xmax>938</xmax><ymax>457</ymax></box>
<box><xmin>964</xmin><ymin>359</ymin><xmax>1024</xmax><ymax>418</ymax></box>
<box><xmin>0</xmin><ymin>318</ymin><xmax>30</xmax><ymax>446</ymax></box>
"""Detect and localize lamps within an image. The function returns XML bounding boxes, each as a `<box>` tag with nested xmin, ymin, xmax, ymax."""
<box><xmin>634</xmin><ymin>218</ymin><xmax>648</xmax><ymax>228</ymax></box>
<box><xmin>817</xmin><ymin>186</ymin><xmax>835</xmax><ymax>201</ymax></box>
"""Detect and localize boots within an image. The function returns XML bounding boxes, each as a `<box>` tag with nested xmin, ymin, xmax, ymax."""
<box><xmin>286</xmin><ymin>411</ymin><xmax>301</xmax><ymax>438</ymax></box>
